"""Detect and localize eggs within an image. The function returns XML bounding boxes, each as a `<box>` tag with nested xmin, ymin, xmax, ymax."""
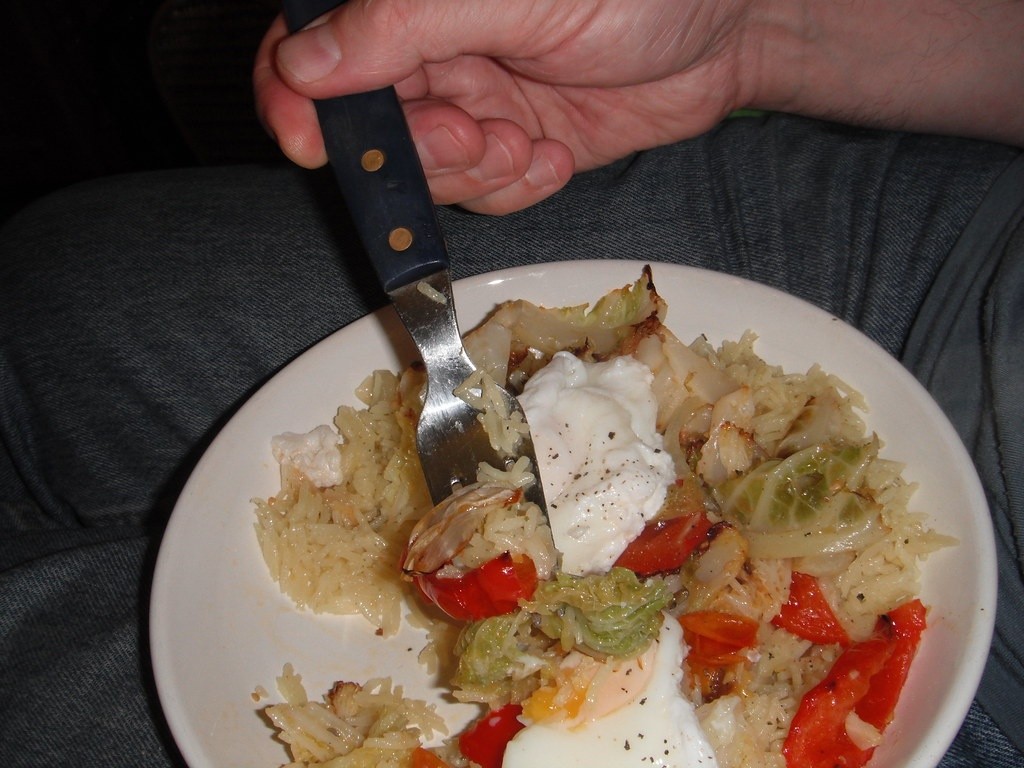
<box><xmin>502</xmin><ymin>350</ymin><xmax>675</xmax><ymax>578</ymax></box>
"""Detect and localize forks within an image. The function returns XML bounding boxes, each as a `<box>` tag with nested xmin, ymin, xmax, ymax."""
<box><xmin>277</xmin><ymin>1</ymin><xmax>554</xmax><ymax>546</ymax></box>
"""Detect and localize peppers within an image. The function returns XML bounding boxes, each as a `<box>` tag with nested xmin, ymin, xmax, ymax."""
<box><xmin>412</xmin><ymin>508</ymin><xmax>929</xmax><ymax>768</ymax></box>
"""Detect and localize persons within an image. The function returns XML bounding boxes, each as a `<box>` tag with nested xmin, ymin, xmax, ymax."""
<box><xmin>0</xmin><ymin>1</ymin><xmax>1024</xmax><ymax>768</ymax></box>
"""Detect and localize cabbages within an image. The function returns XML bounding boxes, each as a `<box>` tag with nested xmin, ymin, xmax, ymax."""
<box><xmin>398</xmin><ymin>266</ymin><xmax>898</xmax><ymax>713</ymax></box>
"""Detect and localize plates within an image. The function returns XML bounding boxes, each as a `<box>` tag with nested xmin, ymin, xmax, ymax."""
<box><xmin>142</xmin><ymin>255</ymin><xmax>1001</xmax><ymax>767</ymax></box>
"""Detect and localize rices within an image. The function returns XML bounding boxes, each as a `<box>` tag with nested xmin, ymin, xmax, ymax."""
<box><xmin>253</xmin><ymin>328</ymin><xmax>961</xmax><ymax>768</ymax></box>
